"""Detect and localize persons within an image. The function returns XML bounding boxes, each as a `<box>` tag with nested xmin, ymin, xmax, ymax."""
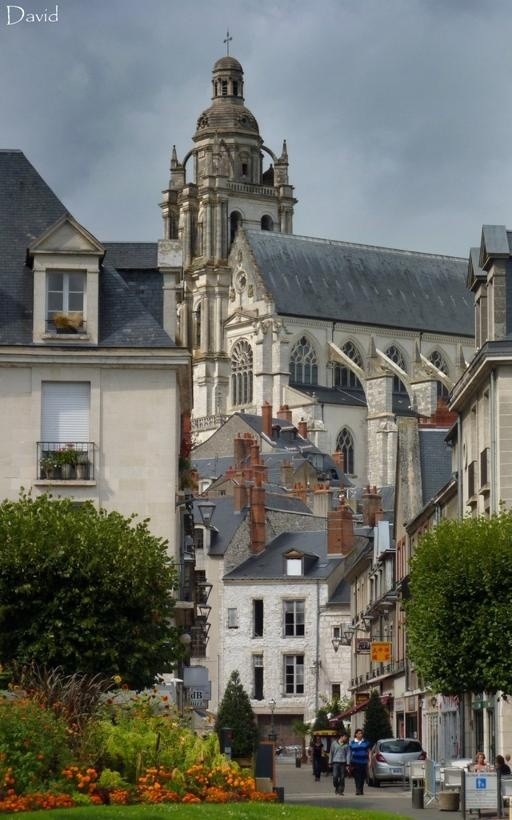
<box><xmin>495</xmin><ymin>755</ymin><xmax>511</xmax><ymax>807</ymax></box>
<box><xmin>349</xmin><ymin>729</ymin><xmax>371</xmax><ymax>795</ymax></box>
<box><xmin>310</xmin><ymin>734</ymin><xmax>325</xmax><ymax>783</ymax></box>
<box><xmin>468</xmin><ymin>752</ymin><xmax>494</xmax><ymax>772</ymax></box>
<box><xmin>328</xmin><ymin>733</ymin><xmax>350</xmax><ymax>795</ymax></box>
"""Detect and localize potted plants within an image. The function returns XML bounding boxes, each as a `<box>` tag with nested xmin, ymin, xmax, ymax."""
<box><xmin>39</xmin><ymin>447</ymin><xmax>89</xmax><ymax>481</ymax></box>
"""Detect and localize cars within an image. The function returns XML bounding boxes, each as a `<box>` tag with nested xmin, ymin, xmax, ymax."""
<box><xmin>366</xmin><ymin>737</ymin><xmax>427</xmax><ymax>787</ymax></box>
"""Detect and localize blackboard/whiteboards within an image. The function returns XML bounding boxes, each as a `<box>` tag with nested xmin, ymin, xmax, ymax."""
<box><xmin>255</xmin><ymin>741</ymin><xmax>276</xmax><ymax>781</ymax></box>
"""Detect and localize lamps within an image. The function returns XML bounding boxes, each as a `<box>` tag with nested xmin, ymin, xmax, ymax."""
<box><xmin>332</xmin><ymin>626</ymin><xmax>368</xmax><ymax>652</ymax></box>
<box><xmin>175</xmin><ymin>496</ymin><xmax>217</xmax><ymax>530</ymax></box>
<box><xmin>179</xmin><ymin>580</ymin><xmax>213</xmax><ymax>650</ymax></box>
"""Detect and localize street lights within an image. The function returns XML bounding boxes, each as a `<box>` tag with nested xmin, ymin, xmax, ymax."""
<box><xmin>268</xmin><ymin>698</ymin><xmax>276</xmax><ymax>741</ymax></box>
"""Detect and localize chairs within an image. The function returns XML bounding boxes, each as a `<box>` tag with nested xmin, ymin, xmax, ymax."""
<box><xmin>404</xmin><ymin>758</ymin><xmax>512</xmax><ymax>820</ymax></box>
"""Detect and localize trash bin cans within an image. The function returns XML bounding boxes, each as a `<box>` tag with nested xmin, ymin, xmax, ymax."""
<box><xmin>410</xmin><ymin>775</ymin><xmax>425</xmax><ymax>810</ymax></box>
